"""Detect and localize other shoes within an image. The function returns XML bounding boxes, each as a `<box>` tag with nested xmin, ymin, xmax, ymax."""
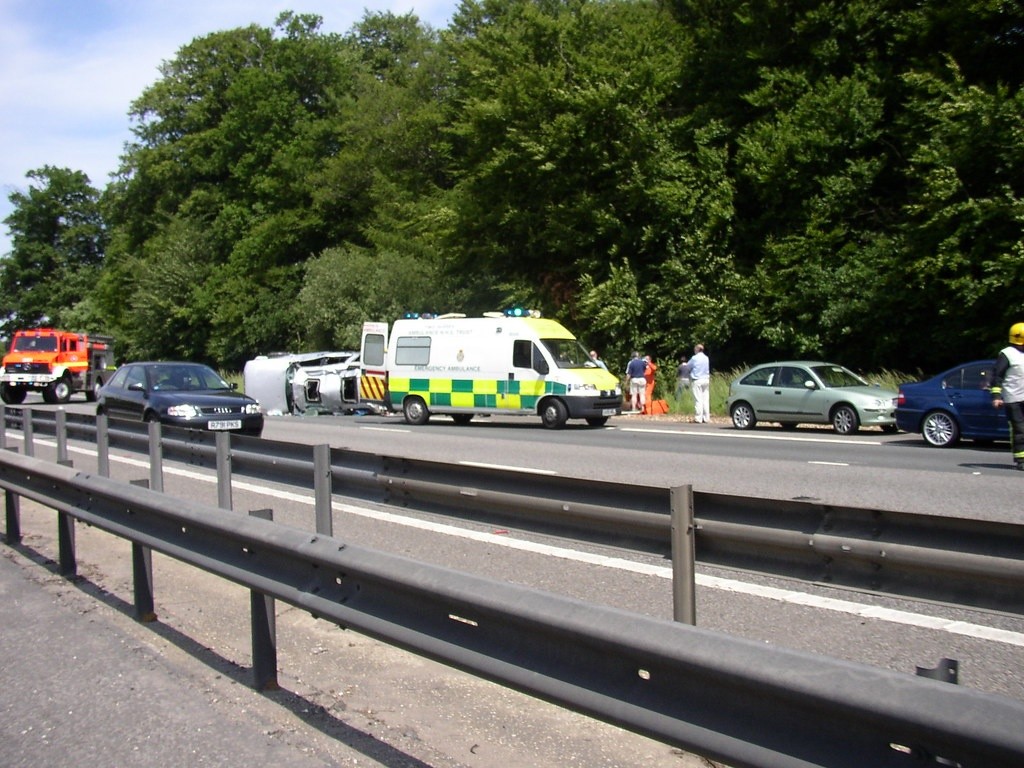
<box><xmin>690</xmin><ymin>419</ymin><xmax>698</xmax><ymax>423</ymax></box>
<box><xmin>1018</xmin><ymin>462</ymin><xmax>1024</xmax><ymax>471</ymax></box>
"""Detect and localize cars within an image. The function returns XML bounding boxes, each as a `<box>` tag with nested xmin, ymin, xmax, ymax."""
<box><xmin>726</xmin><ymin>360</ymin><xmax>899</xmax><ymax>435</ymax></box>
<box><xmin>893</xmin><ymin>360</ymin><xmax>1010</xmax><ymax>449</ymax></box>
<box><xmin>244</xmin><ymin>349</ymin><xmax>388</xmax><ymax>416</ymax></box>
<box><xmin>96</xmin><ymin>361</ymin><xmax>264</xmax><ymax>436</ymax></box>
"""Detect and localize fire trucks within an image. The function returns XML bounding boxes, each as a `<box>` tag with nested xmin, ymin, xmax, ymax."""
<box><xmin>0</xmin><ymin>327</ymin><xmax>117</xmax><ymax>404</ymax></box>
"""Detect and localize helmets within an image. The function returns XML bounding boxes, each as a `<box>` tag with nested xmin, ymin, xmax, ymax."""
<box><xmin>1009</xmin><ymin>323</ymin><xmax>1024</xmax><ymax>345</ymax></box>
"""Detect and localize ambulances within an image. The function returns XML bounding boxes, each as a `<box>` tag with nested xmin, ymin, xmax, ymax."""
<box><xmin>361</xmin><ymin>307</ymin><xmax>623</xmax><ymax>430</ymax></box>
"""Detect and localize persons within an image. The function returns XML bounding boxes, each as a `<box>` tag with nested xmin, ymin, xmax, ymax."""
<box><xmin>991</xmin><ymin>322</ymin><xmax>1024</xmax><ymax>470</ymax></box>
<box><xmin>685</xmin><ymin>344</ymin><xmax>710</xmax><ymax>423</ymax></box>
<box><xmin>560</xmin><ymin>351</ymin><xmax>574</xmax><ymax>364</ymax></box>
<box><xmin>134</xmin><ymin>369</ymin><xmax>161</xmax><ymax>389</ymax></box>
<box><xmin>677</xmin><ymin>357</ymin><xmax>689</xmax><ymax>400</ymax></box>
<box><xmin>585</xmin><ymin>351</ymin><xmax>607</xmax><ymax>370</ymax></box>
<box><xmin>644</xmin><ymin>356</ymin><xmax>657</xmax><ymax>415</ymax></box>
<box><xmin>625</xmin><ymin>351</ymin><xmax>648</xmax><ymax>411</ymax></box>
